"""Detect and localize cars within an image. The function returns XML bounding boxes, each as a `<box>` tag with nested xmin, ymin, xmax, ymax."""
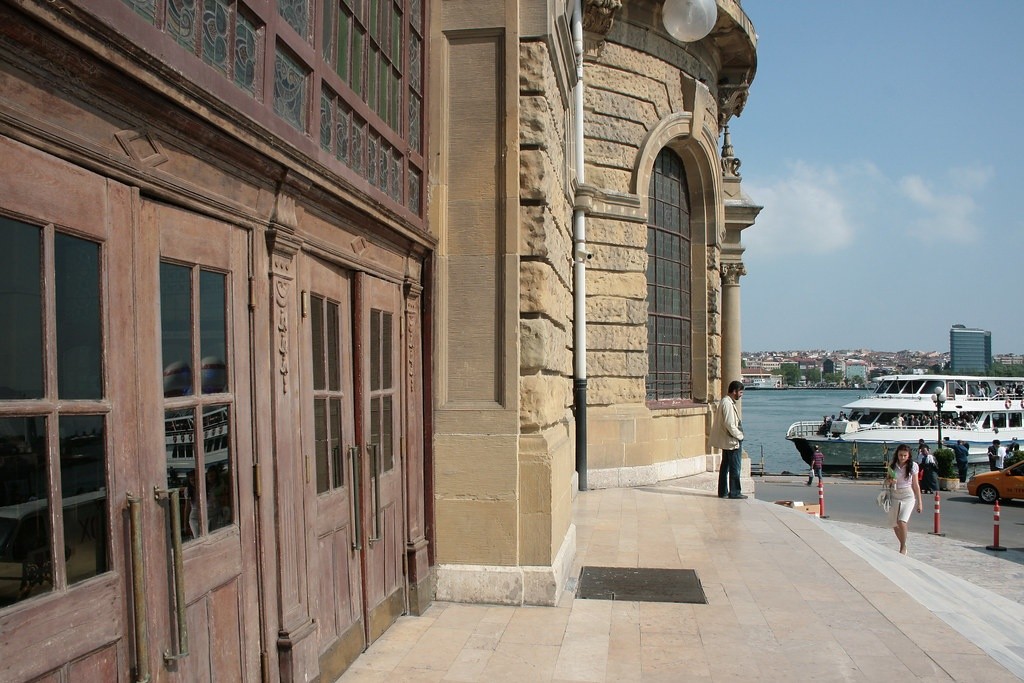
<box><xmin>968</xmin><ymin>459</ymin><xmax>1024</xmax><ymax>504</ymax></box>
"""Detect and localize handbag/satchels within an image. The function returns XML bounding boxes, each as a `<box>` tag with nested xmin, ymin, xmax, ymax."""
<box><xmin>809</xmin><ymin>468</ymin><xmax>814</xmax><ymax>477</ymax></box>
<box><xmin>881</xmin><ymin>484</ymin><xmax>891</xmax><ymax>513</ymax></box>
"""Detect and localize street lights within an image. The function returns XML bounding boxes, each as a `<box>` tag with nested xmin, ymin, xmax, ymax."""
<box><xmin>930</xmin><ymin>385</ymin><xmax>947</xmax><ymax>447</ymax></box>
<box><xmin>820</xmin><ymin>368</ymin><xmax>823</xmax><ymax>387</ymax></box>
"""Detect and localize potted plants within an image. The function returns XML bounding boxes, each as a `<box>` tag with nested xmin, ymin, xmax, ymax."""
<box><xmin>933</xmin><ymin>448</ymin><xmax>960</xmax><ymax>490</ymax></box>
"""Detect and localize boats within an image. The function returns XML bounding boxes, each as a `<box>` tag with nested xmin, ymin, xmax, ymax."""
<box><xmin>785</xmin><ymin>376</ymin><xmax>1024</xmax><ymax>472</ymax></box>
<box><xmin>165</xmin><ymin>407</ymin><xmax>232</xmax><ymax>483</ymax></box>
<box><xmin>744</xmin><ymin>364</ymin><xmax>788</xmax><ymax>390</ymax></box>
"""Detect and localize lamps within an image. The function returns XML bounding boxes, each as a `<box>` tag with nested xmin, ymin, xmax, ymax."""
<box><xmin>663</xmin><ymin>0</ymin><xmax>718</xmax><ymax>43</ymax></box>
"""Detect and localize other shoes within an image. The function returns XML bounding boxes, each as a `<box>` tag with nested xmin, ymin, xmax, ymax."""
<box><xmin>719</xmin><ymin>494</ymin><xmax>729</xmax><ymax>498</ymax></box>
<box><xmin>728</xmin><ymin>494</ymin><xmax>748</xmax><ymax>498</ymax></box>
<box><xmin>925</xmin><ymin>491</ymin><xmax>928</xmax><ymax>494</ymax></box>
<box><xmin>900</xmin><ymin>548</ymin><xmax>908</xmax><ymax>555</ymax></box>
<box><xmin>930</xmin><ymin>492</ymin><xmax>933</xmax><ymax>494</ymax></box>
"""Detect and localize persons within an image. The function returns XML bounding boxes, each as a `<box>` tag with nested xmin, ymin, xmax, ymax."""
<box><xmin>996</xmin><ymin>384</ymin><xmax>1024</xmax><ymax>400</ymax></box>
<box><xmin>988</xmin><ymin>436</ymin><xmax>1020</xmax><ymax>472</ymax></box>
<box><xmin>819</xmin><ymin>410</ymin><xmax>850</xmax><ymax>439</ymax></box>
<box><xmin>806</xmin><ymin>444</ymin><xmax>823</xmax><ymax>488</ymax></box>
<box><xmin>882</xmin><ymin>445</ymin><xmax>922</xmax><ymax>555</ymax></box>
<box><xmin>890</xmin><ymin>411</ymin><xmax>979</xmax><ymax>431</ymax></box>
<box><xmin>916</xmin><ymin>439</ymin><xmax>940</xmax><ymax>493</ymax></box>
<box><xmin>942</xmin><ymin>439</ymin><xmax>970</xmax><ymax>483</ymax></box>
<box><xmin>708</xmin><ymin>381</ymin><xmax>749</xmax><ymax>499</ymax></box>
